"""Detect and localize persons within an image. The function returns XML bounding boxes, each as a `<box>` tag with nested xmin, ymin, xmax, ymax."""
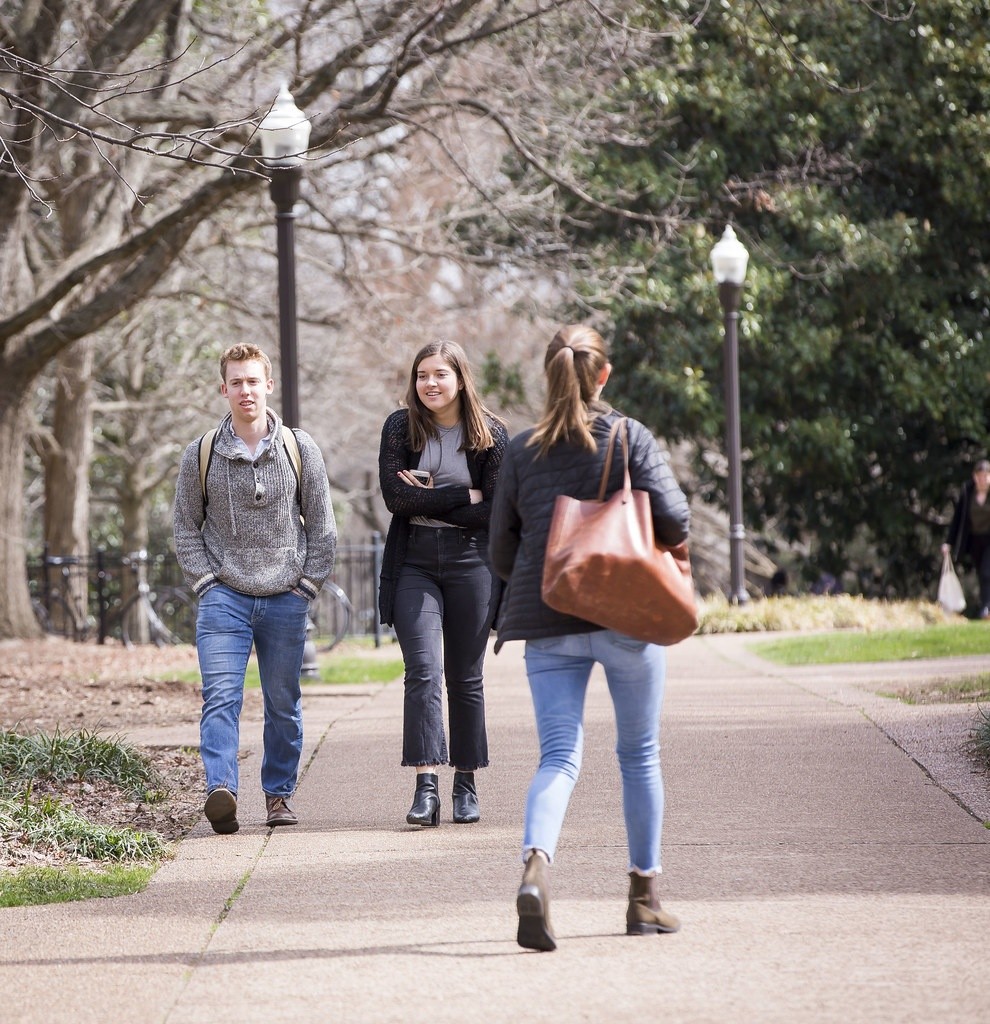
<box><xmin>171</xmin><ymin>344</ymin><xmax>338</xmax><ymax>833</ymax></box>
<box><xmin>486</xmin><ymin>321</ymin><xmax>693</xmax><ymax>954</ymax></box>
<box><xmin>940</xmin><ymin>454</ymin><xmax>990</xmax><ymax>622</ymax></box>
<box><xmin>375</xmin><ymin>340</ymin><xmax>514</xmax><ymax>826</ymax></box>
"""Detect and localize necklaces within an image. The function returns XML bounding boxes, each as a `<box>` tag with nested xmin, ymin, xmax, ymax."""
<box><xmin>432</xmin><ymin>413</ymin><xmax>463</xmax><ymax>444</ymax></box>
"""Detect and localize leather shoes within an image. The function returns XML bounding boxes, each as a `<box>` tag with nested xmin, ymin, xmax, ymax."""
<box><xmin>451</xmin><ymin>771</ymin><xmax>479</xmax><ymax>822</ymax></box>
<box><xmin>203</xmin><ymin>790</ymin><xmax>239</xmax><ymax>833</ymax></box>
<box><xmin>406</xmin><ymin>775</ymin><xmax>442</xmax><ymax>828</ymax></box>
<box><xmin>264</xmin><ymin>794</ymin><xmax>296</xmax><ymax>824</ymax></box>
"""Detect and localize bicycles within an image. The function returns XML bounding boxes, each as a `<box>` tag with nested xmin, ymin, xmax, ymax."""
<box><xmin>23</xmin><ymin>548</ymin><xmax>358</xmax><ymax>657</ymax></box>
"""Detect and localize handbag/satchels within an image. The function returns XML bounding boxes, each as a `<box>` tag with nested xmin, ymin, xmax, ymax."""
<box><xmin>540</xmin><ymin>492</ymin><xmax>698</xmax><ymax>646</ymax></box>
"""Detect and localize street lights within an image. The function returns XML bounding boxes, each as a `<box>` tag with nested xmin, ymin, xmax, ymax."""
<box><xmin>259</xmin><ymin>86</ymin><xmax>319</xmax><ymax>676</ymax></box>
<box><xmin>705</xmin><ymin>226</ymin><xmax>758</xmax><ymax>609</ymax></box>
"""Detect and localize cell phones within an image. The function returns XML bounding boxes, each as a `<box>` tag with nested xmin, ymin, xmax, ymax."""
<box><xmin>409</xmin><ymin>469</ymin><xmax>430</xmax><ymax>487</ymax></box>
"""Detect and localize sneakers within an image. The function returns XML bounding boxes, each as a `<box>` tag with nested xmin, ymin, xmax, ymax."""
<box><xmin>518</xmin><ymin>858</ymin><xmax>557</xmax><ymax>948</ymax></box>
<box><xmin>628</xmin><ymin>901</ymin><xmax>683</xmax><ymax>933</ymax></box>
